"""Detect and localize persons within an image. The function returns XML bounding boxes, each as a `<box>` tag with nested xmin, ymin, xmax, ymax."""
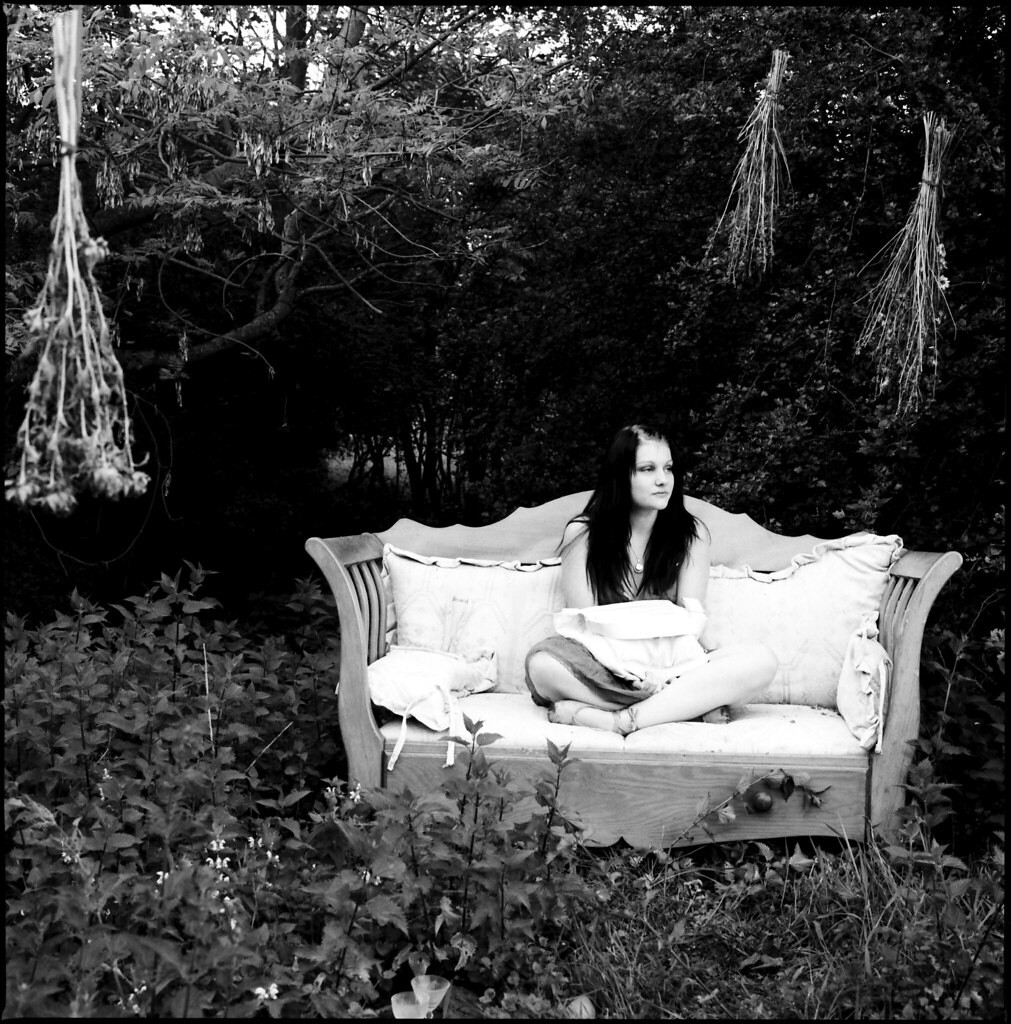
<box><xmin>524</xmin><ymin>426</ymin><xmax>777</xmax><ymax>735</ymax></box>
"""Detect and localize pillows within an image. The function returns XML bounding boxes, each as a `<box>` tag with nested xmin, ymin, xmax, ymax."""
<box><xmin>380</xmin><ymin>534</ymin><xmax>906</xmax><ymax>706</ymax></box>
<box><xmin>836</xmin><ymin>611</ymin><xmax>896</xmax><ymax>756</ymax></box>
<box><xmin>333</xmin><ymin>643</ymin><xmax>497</xmax><ymax>772</ymax></box>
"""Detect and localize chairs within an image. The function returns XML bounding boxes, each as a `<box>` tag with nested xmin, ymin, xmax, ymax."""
<box><xmin>304</xmin><ymin>488</ymin><xmax>964</xmax><ymax>855</ymax></box>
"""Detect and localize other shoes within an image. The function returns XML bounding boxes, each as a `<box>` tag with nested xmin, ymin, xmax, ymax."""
<box><xmin>547</xmin><ymin>699</ymin><xmax>639</xmax><ymax>735</ymax></box>
<box><xmin>703</xmin><ymin>705</ymin><xmax>732</xmax><ymax>724</ymax></box>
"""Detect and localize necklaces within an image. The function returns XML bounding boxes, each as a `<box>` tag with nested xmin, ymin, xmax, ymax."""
<box><xmin>631</xmin><ymin>541</ymin><xmax>645</xmax><ymax>574</ymax></box>
